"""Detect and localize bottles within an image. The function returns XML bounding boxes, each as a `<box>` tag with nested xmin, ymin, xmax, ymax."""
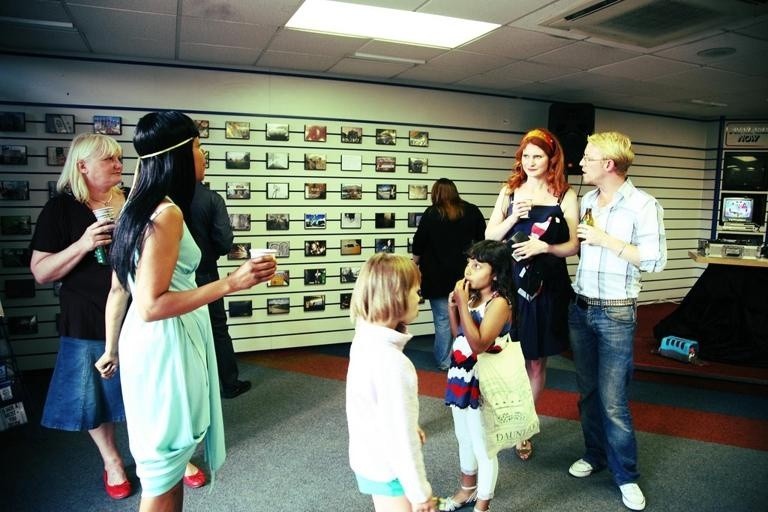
<box><xmin>576</xmin><ymin>207</ymin><xmax>594</xmax><ymax>242</ymax></box>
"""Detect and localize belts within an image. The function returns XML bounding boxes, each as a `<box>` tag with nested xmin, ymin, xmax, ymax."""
<box><xmin>569</xmin><ymin>295</ymin><xmax>639</xmax><ymax>309</ymax></box>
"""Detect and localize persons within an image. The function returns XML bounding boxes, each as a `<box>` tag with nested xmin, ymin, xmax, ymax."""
<box><xmin>94</xmin><ymin>112</ymin><xmax>277</xmax><ymax>511</ymax></box>
<box><xmin>28</xmin><ymin>134</ymin><xmax>206</xmax><ymax>500</ymax></box>
<box><xmin>184</xmin><ymin>184</ymin><xmax>250</xmax><ymax>397</ymax></box>
<box><xmin>348</xmin><ymin>253</ymin><xmax>433</xmax><ymax>512</ymax></box>
<box><xmin>564</xmin><ymin>133</ymin><xmax>667</xmax><ymax>507</ymax></box>
<box><xmin>440</xmin><ymin>240</ymin><xmax>519</xmax><ymax>512</ymax></box>
<box><xmin>413</xmin><ymin>177</ymin><xmax>486</xmax><ymax>373</ymax></box>
<box><xmin>310</xmin><ymin>243</ymin><xmax>324</xmax><ymax>254</ymax></box>
<box><xmin>483</xmin><ymin>128</ymin><xmax>576</xmax><ymax>454</ymax></box>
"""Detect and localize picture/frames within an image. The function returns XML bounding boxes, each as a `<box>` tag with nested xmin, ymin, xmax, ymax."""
<box><xmin>408</xmin><ymin>214</ymin><xmax>422</xmax><ymax>225</ymax></box>
<box><xmin>227</xmin><ymin>183</ymin><xmax>252</xmax><ymax>199</ymax></box>
<box><xmin>267</xmin><ymin>183</ymin><xmax>289</xmax><ymax>198</ymax></box>
<box><xmin>376</xmin><ymin>129</ymin><xmax>398</xmax><ymax>144</ymax></box>
<box><xmin>2</xmin><ymin>111</ymin><xmax>210</xmax><ymax>339</ymax></box>
<box><xmin>409</xmin><ymin>157</ymin><xmax>427</xmax><ymax>172</ymax></box>
<box><xmin>267</xmin><ymin>123</ymin><xmax>289</xmax><ymax>139</ymax></box>
<box><xmin>409</xmin><ymin>132</ymin><xmax>429</xmax><ymax>146</ymax></box>
<box><xmin>340</xmin><ymin>212</ymin><xmax>360</xmax><ymax>228</ymax></box>
<box><xmin>230</xmin><ymin>214</ymin><xmax>250</xmax><ymax>230</ymax></box>
<box><xmin>304</xmin><ymin>213</ymin><xmax>325</xmax><ymax>228</ymax></box>
<box><xmin>228</xmin><ymin>238</ymin><xmax>416</xmax><ymax>317</ymax></box>
<box><xmin>304</xmin><ymin>184</ymin><xmax>326</xmax><ymax>199</ymax></box>
<box><xmin>342</xmin><ymin>127</ymin><xmax>362</xmax><ymax>142</ymax></box>
<box><xmin>376</xmin><ymin>185</ymin><xmax>397</xmax><ymax>199</ymax></box>
<box><xmin>304</xmin><ymin>153</ymin><xmax>327</xmax><ymax>170</ymax></box>
<box><xmin>341</xmin><ymin>184</ymin><xmax>362</xmax><ymax>199</ymax></box>
<box><xmin>341</xmin><ymin>154</ymin><xmax>362</xmax><ymax>170</ymax></box>
<box><xmin>304</xmin><ymin>124</ymin><xmax>326</xmax><ymax>140</ymax></box>
<box><xmin>266</xmin><ymin>153</ymin><xmax>289</xmax><ymax>168</ymax></box>
<box><xmin>267</xmin><ymin>215</ymin><xmax>290</xmax><ymax>229</ymax></box>
<box><xmin>226</xmin><ymin>151</ymin><xmax>250</xmax><ymax>168</ymax></box>
<box><xmin>408</xmin><ymin>184</ymin><xmax>428</xmax><ymax>199</ymax></box>
<box><xmin>375</xmin><ymin>213</ymin><xmax>395</xmax><ymax>228</ymax></box>
<box><xmin>376</xmin><ymin>157</ymin><xmax>395</xmax><ymax>172</ymax></box>
<box><xmin>225</xmin><ymin>122</ymin><xmax>249</xmax><ymax>140</ymax></box>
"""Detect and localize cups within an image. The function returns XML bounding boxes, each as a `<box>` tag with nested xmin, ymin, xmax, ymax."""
<box><xmin>250</xmin><ymin>247</ymin><xmax>278</xmax><ymax>262</ymax></box>
<box><xmin>515</xmin><ymin>195</ymin><xmax>534</xmax><ymax>220</ymax></box>
<box><xmin>92</xmin><ymin>206</ymin><xmax>116</xmax><ymax>234</ymax></box>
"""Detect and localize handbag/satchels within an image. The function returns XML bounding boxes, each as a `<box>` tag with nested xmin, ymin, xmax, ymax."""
<box><xmin>475</xmin><ymin>341</ymin><xmax>543</xmax><ymax>461</ymax></box>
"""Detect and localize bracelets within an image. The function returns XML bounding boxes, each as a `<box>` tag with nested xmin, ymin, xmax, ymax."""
<box><xmin>617</xmin><ymin>243</ymin><xmax>628</xmax><ymax>257</ymax></box>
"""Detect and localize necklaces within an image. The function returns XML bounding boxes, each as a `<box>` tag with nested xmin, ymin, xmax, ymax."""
<box><xmin>88</xmin><ymin>191</ymin><xmax>114</xmax><ymax>207</ymax></box>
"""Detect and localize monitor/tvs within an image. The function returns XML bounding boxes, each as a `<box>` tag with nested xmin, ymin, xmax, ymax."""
<box><xmin>722</xmin><ymin>197</ymin><xmax>754</xmax><ymax>223</ymax></box>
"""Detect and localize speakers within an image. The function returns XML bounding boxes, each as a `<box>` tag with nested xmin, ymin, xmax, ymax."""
<box><xmin>548</xmin><ymin>102</ymin><xmax>595</xmax><ymax>176</ymax></box>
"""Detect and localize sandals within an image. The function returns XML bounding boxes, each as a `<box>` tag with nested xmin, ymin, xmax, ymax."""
<box><xmin>514</xmin><ymin>439</ymin><xmax>534</xmax><ymax>461</ymax></box>
<box><xmin>438</xmin><ymin>481</ymin><xmax>479</xmax><ymax>511</ymax></box>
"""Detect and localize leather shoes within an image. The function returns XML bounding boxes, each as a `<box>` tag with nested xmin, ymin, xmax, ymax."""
<box><xmin>179</xmin><ymin>463</ymin><xmax>207</xmax><ymax>489</ymax></box>
<box><xmin>102</xmin><ymin>465</ymin><xmax>132</xmax><ymax>500</ymax></box>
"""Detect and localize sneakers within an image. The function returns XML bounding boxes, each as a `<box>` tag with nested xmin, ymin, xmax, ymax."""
<box><xmin>221</xmin><ymin>380</ymin><xmax>252</xmax><ymax>399</ymax></box>
<box><xmin>568</xmin><ymin>458</ymin><xmax>596</xmax><ymax>479</ymax></box>
<box><xmin>619</xmin><ymin>481</ymin><xmax>648</xmax><ymax>511</ymax></box>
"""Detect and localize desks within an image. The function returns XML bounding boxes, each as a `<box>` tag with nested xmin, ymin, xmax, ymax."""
<box><xmin>652</xmin><ymin>246</ymin><xmax>768</xmax><ymax>371</ymax></box>
